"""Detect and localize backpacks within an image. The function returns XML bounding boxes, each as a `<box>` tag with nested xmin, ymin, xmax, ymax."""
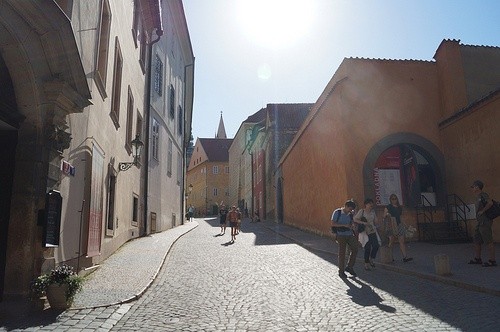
<box><xmin>331</xmin><ymin>209</ymin><xmax>353</xmax><ymax>233</ymax></box>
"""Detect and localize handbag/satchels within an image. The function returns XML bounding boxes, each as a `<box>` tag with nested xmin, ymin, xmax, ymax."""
<box><xmin>373</xmin><ymin>214</ymin><xmax>380</xmax><ymax>233</ymax></box>
<box><xmin>358</xmin><ymin>209</ymin><xmax>367</xmax><ymax>233</ymax></box>
<box><xmin>481</xmin><ymin>193</ymin><xmax>500</xmax><ymax>219</ymax></box>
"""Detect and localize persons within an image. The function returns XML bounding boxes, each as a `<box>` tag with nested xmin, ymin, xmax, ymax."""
<box><xmin>255</xmin><ymin>209</ymin><xmax>258</xmax><ymax>222</ymax></box>
<box><xmin>225</xmin><ymin>210</ymin><xmax>228</xmax><ymax>214</ymax></box>
<box><xmin>228</xmin><ymin>206</ymin><xmax>237</xmax><ymax>243</ymax></box>
<box><xmin>220</xmin><ymin>209</ymin><xmax>226</xmax><ymax>235</ymax></box>
<box><xmin>354</xmin><ymin>199</ymin><xmax>379</xmax><ymax>271</ymax></box>
<box><xmin>331</xmin><ymin>200</ymin><xmax>359</xmax><ymax>278</ymax></box>
<box><xmin>235</xmin><ymin>208</ymin><xmax>240</xmax><ymax>235</ymax></box>
<box><xmin>188</xmin><ymin>204</ymin><xmax>193</xmax><ymax>222</ymax></box>
<box><xmin>382</xmin><ymin>194</ymin><xmax>413</xmax><ymax>262</ymax></box>
<box><xmin>228</xmin><ymin>209</ymin><xmax>231</xmax><ymax>213</ymax></box>
<box><xmin>467</xmin><ymin>181</ymin><xmax>496</xmax><ymax>266</ymax></box>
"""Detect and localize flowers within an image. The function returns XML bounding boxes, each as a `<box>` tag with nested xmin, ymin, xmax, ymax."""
<box><xmin>31</xmin><ymin>263</ymin><xmax>87</xmax><ymax>309</ymax></box>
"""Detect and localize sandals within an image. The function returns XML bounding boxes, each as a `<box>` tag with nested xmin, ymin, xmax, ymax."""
<box><xmin>467</xmin><ymin>257</ymin><xmax>482</xmax><ymax>264</ymax></box>
<box><xmin>481</xmin><ymin>259</ymin><xmax>498</xmax><ymax>267</ymax></box>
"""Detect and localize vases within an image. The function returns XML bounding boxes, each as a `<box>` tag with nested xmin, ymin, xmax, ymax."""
<box><xmin>43</xmin><ymin>283</ymin><xmax>68</xmax><ymax>309</ymax></box>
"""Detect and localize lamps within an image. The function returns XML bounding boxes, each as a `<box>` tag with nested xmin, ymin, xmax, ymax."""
<box><xmin>118</xmin><ymin>133</ymin><xmax>145</xmax><ymax>172</ymax></box>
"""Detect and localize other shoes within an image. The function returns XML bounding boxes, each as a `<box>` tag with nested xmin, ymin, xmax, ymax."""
<box><xmin>386</xmin><ymin>256</ymin><xmax>395</xmax><ymax>262</ymax></box>
<box><xmin>370</xmin><ymin>257</ymin><xmax>376</xmax><ymax>266</ymax></box>
<box><xmin>345</xmin><ymin>265</ymin><xmax>356</xmax><ymax>276</ymax></box>
<box><xmin>363</xmin><ymin>261</ymin><xmax>371</xmax><ymax>270</ymax></box>
<box><xmin>401</xmin><ymin>256</ymin><xmax>411</xmax><ymax>262</ymax></box>
<box><xmin>338</xmin><ymin>270</ymin><xmax>347</xmax><ymax>278</ymax></box>
